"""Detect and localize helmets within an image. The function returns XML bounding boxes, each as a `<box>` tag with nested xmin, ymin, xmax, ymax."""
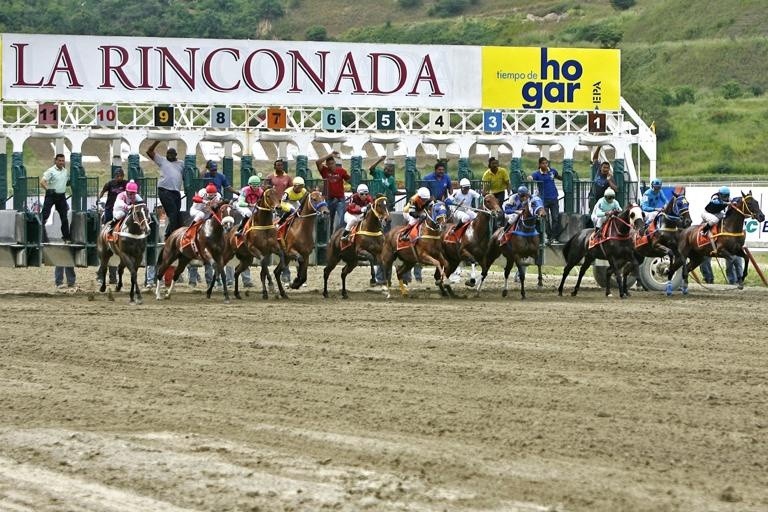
<box><xmin>719</xmin><ymin>186</ymin><xmax>729</xmax><ymax>195</ymax></box>
<box><xmin>357</xmin><ymin>183</ymin><xmax>368</xmax><ymax>193</ymax></box>
<box><xmin>206</xmin><ymin>183</ymin><xmax>217</xmax><ymax>193</ymax></box>
<box><xmin>418</xmin><ymin>187</ymin><xmax>430</xmax><ymax>199</ymax></box>
<box><xmin>126</xmin><ymin>179</ymin><xmax>138</xmax><ymax>192</ymax></box>
<box><xmin>651</xmin><ymin>179</ymin><xmax>662</xmax><ymax>186</ymax></box>
<box><xmin>248</xmin><ymin>175</ymin><xmax>261</xmax><ymax>186</ymax></box>
<box><xmin>292</xmin><ymin>176</ymin><xmax>305</xmax><ymax>186</ymax></box>
<box><xmin>604</xmin><ymin>187</ymin><xmax>616</xmax><ymax>196</ymax></box>
<box><xmin>460</xmin><ymin>178</ymin><xmax>470</xmax><ymax>186</ymax></box>
<box><xmin>518</xmin><ymin>186</ymin><xmax>527</xmax><ymax>195</ymax></box>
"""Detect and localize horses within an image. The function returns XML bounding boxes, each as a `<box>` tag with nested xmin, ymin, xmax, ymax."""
<box><xmin>323</xmin><ymin>190</ymin><xmax>391</xmax><ymax>301</ymax></box>
<box><xmin>155</xmin><ymin>184</ymin><xmax>331</xmax><ymax>304</ymax></box>
<box><xmin>557</xmin><ymin>190</ymin><xmax>765</xmax><ymax>297</ymax></box>
<box><xmin>381</xmin><ymin>193</ymin><xmax>549</xmax><ymax>300</ymax></box>
<box><xmin>96</xmin><ymin>196</ymin><xmax>152</xmax><ymax>307</ymax></box>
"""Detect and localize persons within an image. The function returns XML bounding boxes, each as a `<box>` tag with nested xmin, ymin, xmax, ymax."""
<box><xmin>53</xmin><ymin>201</ymin><xmax>77</xmax><ymax>286</ymax></box>
<box><xmin>588</xmin><ymin>146</ymin><xmax>743</xmax><ymax>285</ymax></box>
<box><xmin>96</xmin><ymin>141</ymin><xmax>564</xmax><ymax>286</ymax></box>
<box><xmin>40</xmin><ymin>153</ymin><xmax>73</xmax><ymax>244</ymax></box>
